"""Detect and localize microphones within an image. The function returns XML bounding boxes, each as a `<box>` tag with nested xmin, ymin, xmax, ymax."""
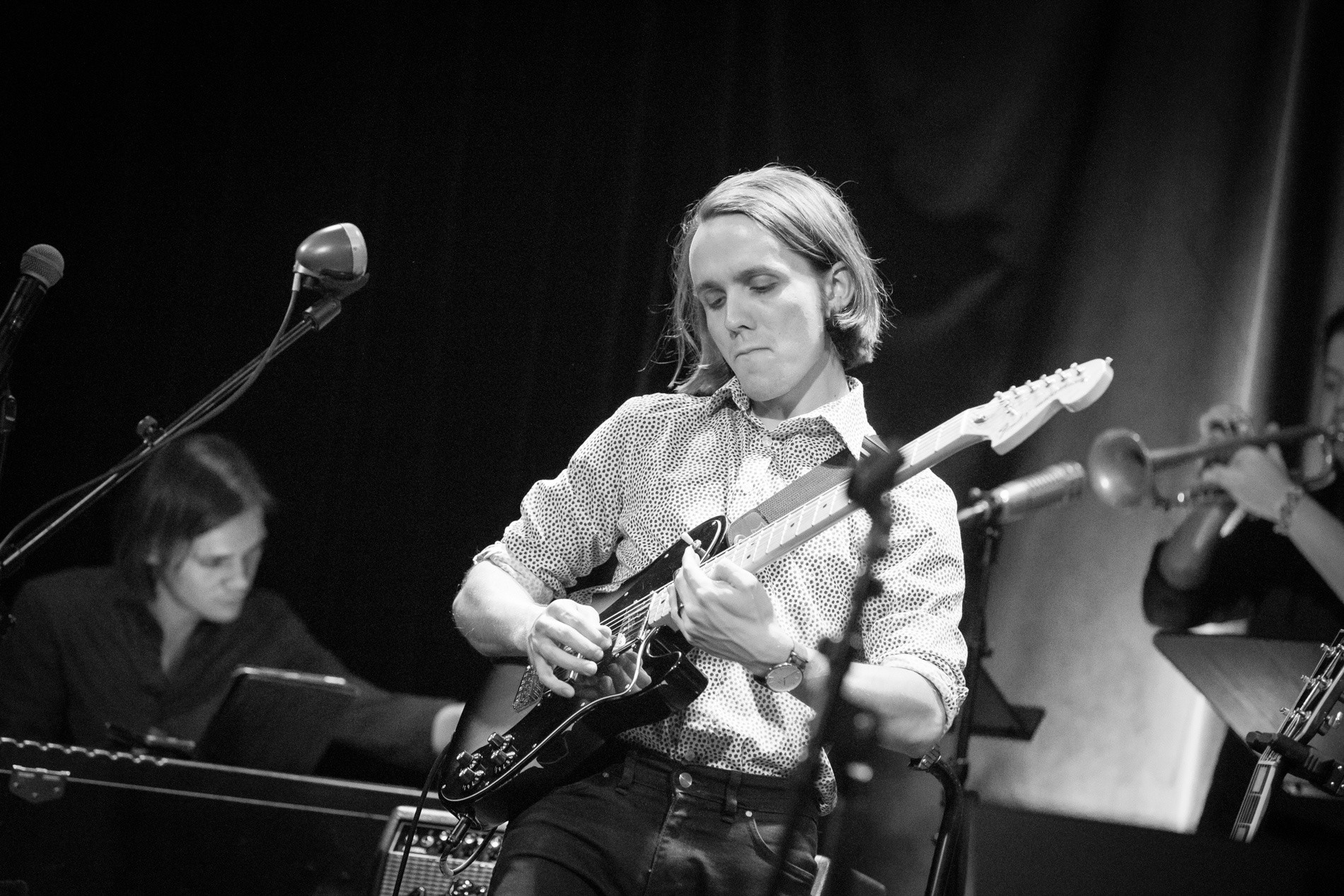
<box><xmin>0</xmin><ymin>243</ymin><xmax>64</xmax><ymax>386</ymax></box>
<box><xmin>956</xmin><ymin>462</ymin><xmax>1086</xmax><ymax>526</ymax></box>
<box><xmin>291</xmin><ymin>223</ymin><xmax>368</xmax><ymax>294</ymax></box>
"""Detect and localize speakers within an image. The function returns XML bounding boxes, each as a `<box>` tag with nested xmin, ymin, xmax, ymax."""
<box><xmin>363</xmin><ymin>806</ymin><xmax>508</xmax><ymax>896</ymax></box>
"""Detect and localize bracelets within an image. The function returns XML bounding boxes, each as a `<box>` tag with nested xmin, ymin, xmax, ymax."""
<box><xmin>1273</xmin><ymin>484</ymin><xmax>1309</xmax><ymax>537</ymax></box>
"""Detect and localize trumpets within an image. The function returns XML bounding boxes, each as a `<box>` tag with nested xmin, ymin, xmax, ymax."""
<box><xmin>1087</xmin><ymin>422</ymin><xmax>1344</xmax><ymax>512</ymax></box>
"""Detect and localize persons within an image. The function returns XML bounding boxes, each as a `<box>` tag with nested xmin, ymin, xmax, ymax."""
<box><xmin>0</xmin><ymin>432</ymin><xmax>472</xmax><ymax>782</ymax></box>
<box><xmin>1142</xmin><ymin>307</ymin><xmax>1344</xmax><ymax>853</ymax></box>
<box><xmin>452</xmin><ymin>164</ymin><xmax>972</xmax><ymax>896</ymax></box>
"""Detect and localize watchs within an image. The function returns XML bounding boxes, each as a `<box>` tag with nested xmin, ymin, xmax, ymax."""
<box><xmin>753</xmin><ymin>641</ymin><xmax>809</xmax><ymax>694</ymax></box>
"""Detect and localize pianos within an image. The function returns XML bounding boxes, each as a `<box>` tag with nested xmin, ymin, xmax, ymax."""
<box><xmin>0</xmin><ymin>665</ymin><xmax>469</xmax><ymax>896</ymax></box>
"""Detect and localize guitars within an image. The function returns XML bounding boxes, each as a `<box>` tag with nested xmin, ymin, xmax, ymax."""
<box><xmin>437</xmin><ymin>356</ymin><xmax>1114</xmax><ymax>831</ymax></box>
